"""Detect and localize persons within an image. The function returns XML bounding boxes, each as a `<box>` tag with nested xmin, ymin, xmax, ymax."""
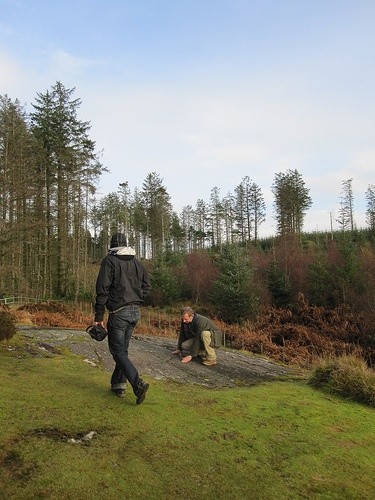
<box><xmin>95</xmin><ymin>233</ymin><xmax>152</xmax><ymax>404</ymax></box>
<box><xmin>172</xmin><ymin>305</ymin><xmax>223</xmax><ymax>365</ymax></box>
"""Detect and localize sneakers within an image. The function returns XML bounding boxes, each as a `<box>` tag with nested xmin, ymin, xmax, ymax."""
<box><xmin>134</xmin><ymin>382</ymin><xmax>149</xmax><ymax>405</ymax></box>
<box><xmin>202</xmin><ymin>359</ymin><xmax>217</xmax><ymax>366</ymax></box>
<box><xmin>108</xmin><ymin>387</ymin><xmax>126</xmax><ymax>398</ymax></box>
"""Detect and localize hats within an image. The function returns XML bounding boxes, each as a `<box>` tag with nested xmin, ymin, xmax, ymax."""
<box><xmin>110</xmin><ymin>233</ymin><xmax>127</xmax><ymax>249</ymax></box>
<box><xmin>86</xmin><ymin>324</ymin><xmax>108</xmax><ymax>342</ymax></box>
